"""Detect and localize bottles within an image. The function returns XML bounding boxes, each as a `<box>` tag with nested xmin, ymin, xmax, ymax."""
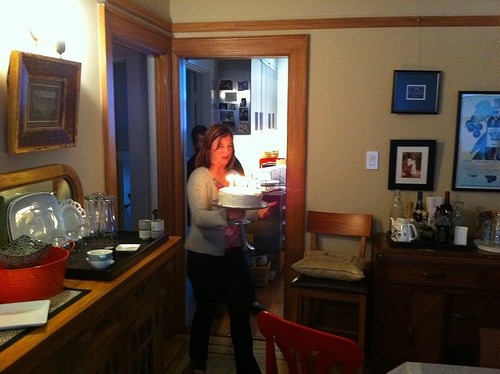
<box><xmin>389</xmin><ymin>190</ymin><xmax>404</xmax><ymax>219</ymax></box>
<box><xmin>405</xmin><ymin>189</ymin><xmax>465</xmax><ymax>245</ymax></box>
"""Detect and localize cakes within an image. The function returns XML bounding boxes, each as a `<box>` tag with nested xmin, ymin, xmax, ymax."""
<box><xmin>218</xmin><ymin>185</ymin><xmax>263</xmax><ymax>209</ymax></box>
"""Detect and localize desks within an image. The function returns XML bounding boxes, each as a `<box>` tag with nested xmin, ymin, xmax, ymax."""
<box><xmin>371</xmin><ymin>232</ymin><xmax>499</xmax><ymax>374</ymax></box>
<box><xmin>0</xmin><ymin>230</ymin><xmax>184</xmax><ymax>373</ymax></box>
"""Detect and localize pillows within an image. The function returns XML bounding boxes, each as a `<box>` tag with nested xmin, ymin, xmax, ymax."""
<box><xmin>292</xmin><ymin>251</ymin><xmax>371</xmax><ymax>282</ymax></box>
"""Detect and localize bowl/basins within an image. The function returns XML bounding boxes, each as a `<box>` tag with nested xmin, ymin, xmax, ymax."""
<box><xmin>0</xmin><ymin>235</ymin><xmax>50</xmax><ymax>268</ymax></box>
<box><xmin>85</xmin><ymin>249</ymin><xmax>114</xmax><ymax>269</ymax></box>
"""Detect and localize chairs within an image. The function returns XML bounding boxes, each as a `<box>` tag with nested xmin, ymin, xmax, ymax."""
<box><xmin>259</xmin><ymin>210</ymin><xmax>374</xmax><ymax>374</ymax></box>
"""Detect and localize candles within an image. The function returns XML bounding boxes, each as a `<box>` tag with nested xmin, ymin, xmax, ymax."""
<box><xmin>225</xmin><ymin>174</ymin><xmax>261</xmax><ymax>190</ymax></box>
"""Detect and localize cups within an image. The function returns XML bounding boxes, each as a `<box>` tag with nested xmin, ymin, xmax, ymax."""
<box><xmin>481</xmin><ymin>220</ymin><xmax>500</xmax><ymax>247</ymax></box>
<box><xmin>454</xmin><ymin>226</ymin><xmax>468</xmax><ymax>245</ymax></box>
<box><xmin>138</xmin><ymin>219</ymin><xmax>164</xmax><ymax>239</ymax></box>
<box><xmin>83</xmin><ymin>192</ymin><xmax>117</xmax><ymax>238</ymax></box>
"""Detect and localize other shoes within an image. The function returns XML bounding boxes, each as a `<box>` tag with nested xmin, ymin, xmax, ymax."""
<box><xmin>191</xmin><ymin>361</ymin><xmax>206</xmax><ymax>374</ymax></box>
<box><xmin>251</xmin><ymin>300</ymin><xmax>264</xmax><ymax>311</ymax></box>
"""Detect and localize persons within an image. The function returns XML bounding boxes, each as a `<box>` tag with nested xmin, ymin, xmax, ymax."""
<box><xmin>187</xmin><ymin>125</ymin><xmax>244</xmax><ymax>227</ymax></box>
<box><xmin>183</xmin><ymin>123</ymin><xmax>262</xmax><ymax>374</ymax></box>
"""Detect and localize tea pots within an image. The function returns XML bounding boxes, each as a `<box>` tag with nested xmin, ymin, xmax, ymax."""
<box><xmin>389</xmin><ymin>217</ymin><xmax>418</xmax><ymax>243</ymax></box>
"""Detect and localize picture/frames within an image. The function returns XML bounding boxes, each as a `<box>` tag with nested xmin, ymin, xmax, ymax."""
<box><xmin>390</xmin><ymin>70</ymin><xmax>442</xmax><ymax>114</ymax></box>
<box><xmin>451</xmin><ymin>90</ymin><xmax>500</xmax><ymax>192</ymax></box>
<box><xmin>388</xmin><ymin>139</ymin><xmax>436</xmax><ymax>191</ymax></box>
<box><xmin>6</xmin><ymin>51</ymin><xmax>82</xmax><ymax>154</ymax></box>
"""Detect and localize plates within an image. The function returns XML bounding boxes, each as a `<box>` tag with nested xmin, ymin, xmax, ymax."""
<box><xmin>5</xmin><ymin>191</ymin><xmax>86</xmax><ymax>246</ymax></box>
<box><xmin>115</xmin><ymin>244</ymin><xmax>141</xmax><ymax>252</ymax></box>
<box><xmin>0</xmin><ymin>299</ymin><xmax>51</xmax><ymax>329</ymax></box>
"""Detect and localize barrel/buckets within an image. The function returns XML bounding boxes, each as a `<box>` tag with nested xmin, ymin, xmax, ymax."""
<box><xmin>0</xmin><ymin>240</ymin><xmax>75</xmax><ymax>303</ymax></box>
